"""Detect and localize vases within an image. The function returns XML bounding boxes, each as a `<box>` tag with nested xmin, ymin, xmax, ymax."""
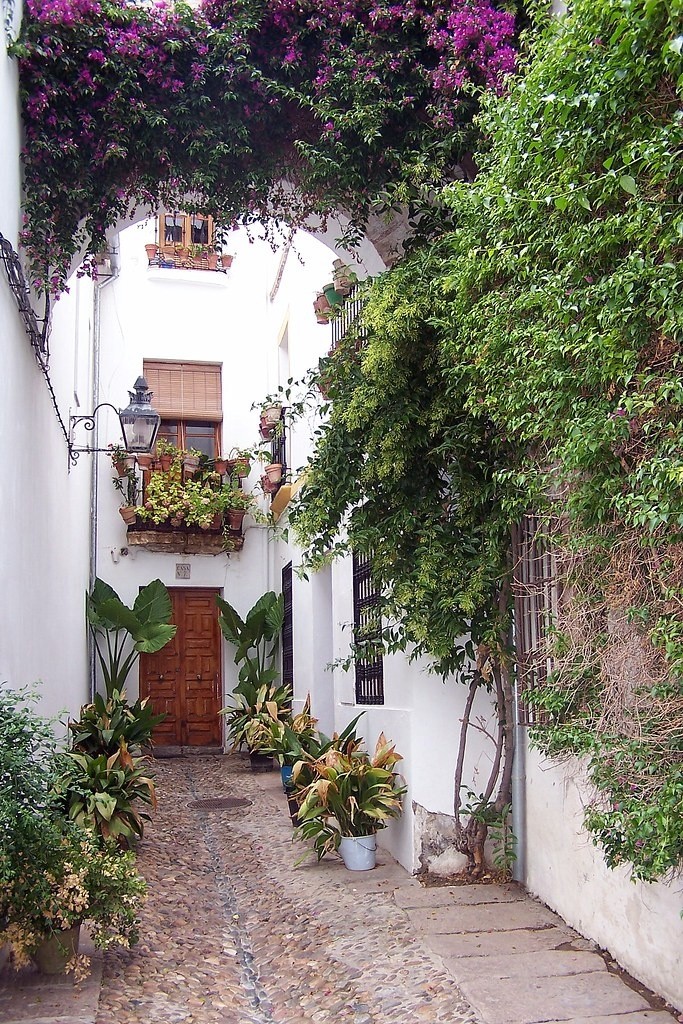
<box><xmin>207</xmin><ymin>255</ymin><xmax>220</xmax><ymax>269</ymax></box>
<box><xmin>322</xmin><ymin>283</ymin><xmax>343</xmax><ymax>306</ymax></box>
<box><xmin>162</xmin><ymin>246</ymin><xmax>176</xmax><ymax>261</ymax></box>
<box><xmin>125</xmin><ymin>455</ymin><xmax>136</xmax><ymax>469</ymax></box>
<box><xmin>138</xmin><ymin>456</ymin><xmax>153</xmax><ymax>471</ymax></box>
<box><xmin>331</xmin><ymin>271</ymin><xmax>350</xmax><ymax>295</ymax></box>
<box><xmin>333</xmin><ymin>260</ymin><xmax>353</xmax><ymax>286</ymax></box>
<box><xmin>317</xmin><ymin>295</ymin><xmax>336</xmax><ymax>315</ymax></box>
<box><xmin>115</xmin><ymin>461</ymin><xmax>125</xmax><ymax>476</ymax></box>
<box><xmin>144</xmin><ymin>243</ymin><xmax>159</xmax><ymax>261</ymax></box>
<box><xmin>260</xmin><ymin>464</ymin><xmax>283</xmax><ymax>494</ymax></box>
<box><xmin>259</xmin><ymin>403</ymin><xmax>283</xmax><ymax>439</ymax></box>
<box><xmin>313</xmin><ymin>301</ymin><xmax>329</xmax><ymax>325</ymax></box>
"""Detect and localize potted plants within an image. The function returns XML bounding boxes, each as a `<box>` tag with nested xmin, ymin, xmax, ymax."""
<box><xmin>287</xmin><ymin>711</ymin><xmax>368</xmax><ymax>828</ymax></box>
<box><xmin>218</xmin><ymin>684</ymin><xmax>294</xmax><ymax>773</ymax></box>
<box><xmin>176</xmin><ymin>242</ymin><xmax>189</xmax><ymax>263</ymax></box>
<box><xmin>257</xmin><ymin>692</ymin><xmax>319</xmax><ymax>787</ymax></box>
<box><xmin>0</xmin><ymin>680</ymin><xmax>152</xmax><ymax>992</ymax></box>
<box><xmin>112</xmin><ymin>437</ymin><xmax>258</xmax><ymax>530</ymax></box>
<box><xmin>287</xmin><ymin>730</ymin><xmax>408</xmax><ymax>871</ymax></box>
<box><xmin>221</xmin><ymin>251</ymin><xmax>238</xmax><ymax>268</ymax></box>
<box><xmin>187</xmin><ymin>242</ymin><xmax>208</xmax><ymax>264</ymax></box>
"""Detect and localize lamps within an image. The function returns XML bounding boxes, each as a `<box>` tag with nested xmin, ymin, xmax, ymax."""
<box><xmin>68</xmin><ymin>376</ymin><xmax>162</xmax><ymax>474</ymax></box>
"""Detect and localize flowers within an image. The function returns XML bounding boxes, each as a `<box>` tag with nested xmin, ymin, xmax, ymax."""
<box><xmin>105</xmin><ymin>443</ymin><xmax>129</xmax><ymax>465</ymax></box>
